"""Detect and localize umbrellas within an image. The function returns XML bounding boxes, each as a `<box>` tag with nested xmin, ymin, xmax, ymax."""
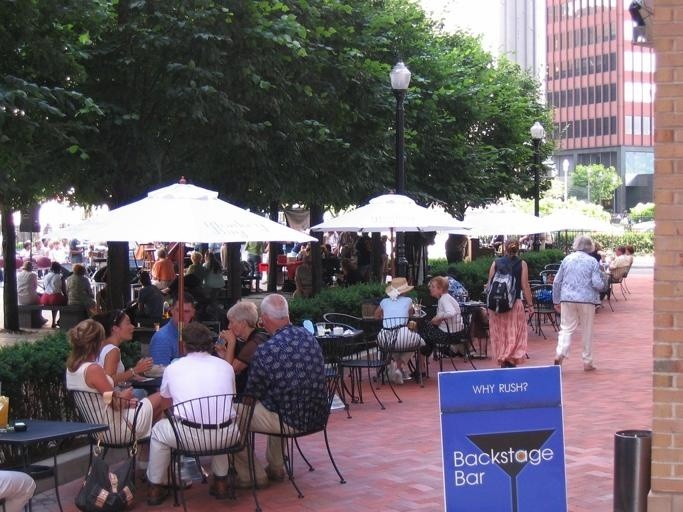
<box><xmin>309</xmin><ymin>189</ymin><xmax>473</xmax><ymax>279</ymax></box>
<box><xmin>540</xmin><ymin>208</ymin><xmax>617</xmax><ymax>256</ymax></box>
<box><xmin>41</xmin><ymin>174</ymin><xmax>319</xmax><ymax>357</ymax></box>
<box><xmin>445</xmin><ymin>202</ymin><xmax>551</xmax><ymax>256</ymax></box>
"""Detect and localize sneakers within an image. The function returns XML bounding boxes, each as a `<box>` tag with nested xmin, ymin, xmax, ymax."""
<box><xmin>554</xmin><ymin>355</ymin><xmax>562</xmax><ymax>366</ymax></box>
<box><xmin>393</xmin><ymin>368</ymin><xmax>402</xmax><ymax>384</ymax></box>
<box><xmin>584</xmin><ymin>364</ymin><xmax>595</xmax><ymax>371</ymax></box>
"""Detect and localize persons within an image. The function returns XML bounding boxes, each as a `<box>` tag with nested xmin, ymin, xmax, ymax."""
<box><xmin>552</xmin><ymin>234</ymin><xmax>612</xmax><ymax>373</ymax></box>
<box><xmin>375</xmin><ymin>274</ymin><xmax>477</xmax><ymax>384</ymax></box>
<box><xmin>1</xmin><ymin>470</ymin><xmax>37</xmax><ymax>510</ymax></box>
<box><xmin>588</xmin><ymin>241</ymin><xmax>634</xmax><ymax>312</ymax></box>
<box><xmin>282</xmin><ymin>230</ymin><xmax>471</xmax><ymax>302</ymax></box>
<box><xmin>0</xmin><ymin>222</ymin><xmax>269</xmax><ymax>340</ymax></box>
<box><xmin>519</xmin><ymin>231</ymin><xmax>553</xmax><ymax>251</ymax></box>
<box><xmin>486</xmin><ymin>238</ymin><xmax>535</xmax><ymax>368</ymax></box>
<box><xmin>66</xmin><ymin>292</ymin><xmax>330</xmax><ymax>503</ymax></box>
<box><xmin>489</xmin><ymin>236</ymin><xmax>507</xmax><ymax>251</ymax></box>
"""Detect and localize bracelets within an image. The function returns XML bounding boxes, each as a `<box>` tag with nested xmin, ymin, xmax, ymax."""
<box><xmin>528</xmin><ymin>304</ymin><xmax>534</xmax><ymax>308</ymax></box>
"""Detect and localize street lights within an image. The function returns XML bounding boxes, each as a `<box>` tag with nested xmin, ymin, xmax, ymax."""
<box><xmin>529</xmin><ymin>121</ymin><xmax>546</xmax><ymax>253</ymax></box>
<box><xmin>562</xmin><ymin>159</ymin><xmax>568</xmax><ymax>201</ymax></box>
<box><xmin>586</xmin><ymin>167</ymin><xmax>591</xmax><ymax>201</ymax></box>
<box><xmin>388</xmin><ymin>61</ymin><xmax>412</xmax><ymax>281</ymax></box>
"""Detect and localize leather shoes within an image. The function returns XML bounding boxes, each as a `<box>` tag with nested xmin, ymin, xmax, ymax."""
<box><xmin>262</xmin><ymin>466</ymin><xmax>286</xmax><ymax>482</ymax></box>
<box><xmin>234</xmin><ymin>475</ymin><xmax>267</xmax><ymax>489</ymax></box>
<box><xmin>409</xmin><ymin>370</ymin><xmax>425</xmax><ymax>377</ymax></box>
<box><xmin>209</xmin><ymin>479</ymin><xmax>227</xmax><ymax>498</ymax></box>
<box><xmin>146</xmin><ymin>486</ymin><xmax>168</xmax><ymax>505</ymax></box>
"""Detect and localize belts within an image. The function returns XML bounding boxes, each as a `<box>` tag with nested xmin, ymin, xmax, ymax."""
<box><xmin>176</xmin><ymin>417</ymin><xmax>232</xmax><ymax>429</ymax></box>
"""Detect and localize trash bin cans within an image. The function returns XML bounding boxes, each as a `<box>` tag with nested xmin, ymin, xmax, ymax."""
<box><xmin>613</xmin><ymin>430</ymin><xmax>652</xmax><ymax>512</ymax></box>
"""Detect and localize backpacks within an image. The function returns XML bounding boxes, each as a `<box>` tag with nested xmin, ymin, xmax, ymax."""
<box><xmin>485</xmin><ymin>257</ymin><xmax>520</xmax><ymax>313</ymax></box>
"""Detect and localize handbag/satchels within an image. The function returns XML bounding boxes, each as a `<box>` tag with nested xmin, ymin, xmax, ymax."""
<box><xmin>73</xmin><ymin>453</ymin><xmax>138</xmax><ymax>511</ymax></box>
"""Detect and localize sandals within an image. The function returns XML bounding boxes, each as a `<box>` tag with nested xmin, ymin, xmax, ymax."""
<box><xmin>169</xmin><ymin>478</ymin><xmax>193</xmax><ymax>490</ymax></box>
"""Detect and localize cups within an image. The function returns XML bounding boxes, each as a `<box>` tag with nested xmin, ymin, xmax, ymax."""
<box><xmin>215</xmin><ymin>334</ymin><xmax>227</xmax><ymax>349</ymax></box>
<box><xmin>0</xmin><ymin>396</ymin><xmax>9</xmax><ymax>434</ymax></box>
<box><xmin>333</xmin><ymin>327</ymin><xmax>344</xmax><ymax>336</ymax></box>
<box><xmin>316</xmin><ymin>322</ymin><xmax>326</xmax><ymax>336</ymax></box>
<box><xmin>414</xmin><ymin>303</ymin><xmax>422</xmax><ymax>317</ymax></box>
<box><xmin>153</xmin><ymin>322</ymin><xmax>160</xmax><ymax>332</ymax></box>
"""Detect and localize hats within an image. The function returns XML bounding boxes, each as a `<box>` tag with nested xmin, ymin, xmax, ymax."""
<box><xmin>385</xmin><ymin>277</ymin><xmax>414</xmax><ymax>301</ymax></box>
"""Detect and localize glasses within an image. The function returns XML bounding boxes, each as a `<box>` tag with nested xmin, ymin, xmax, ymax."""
<box><xmin>112</xmin><ymin>308</ymin><xmax>125</xmax><ymax>324</ymax></box>
